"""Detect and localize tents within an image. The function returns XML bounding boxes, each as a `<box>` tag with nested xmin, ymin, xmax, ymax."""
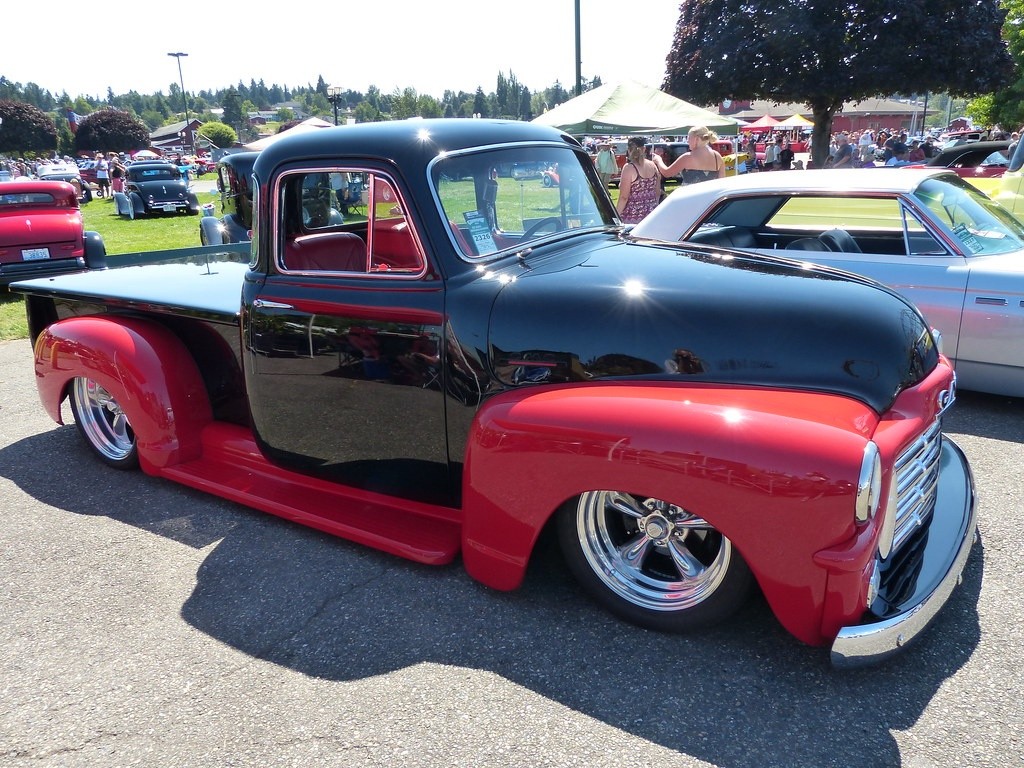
<box><xmin>521</xmin><ymin>80</ymin><xmax>739</xmax><ymax>222</ymax></box>
<box><xmin>771</xmin><ymin>114</ymin><xmax>815</xmax><ymax>143</ymax></box>
<box><xmin>727</xmin><ymin>117</ymin><xmax>751</xmax><ymax>126</ymax></box>
<box><xmin>742</xmin><ymin>114</ymin><xmax>781</xmax><ymax>139</ymax></box>
<box><xmin>241</xmin><ymin>117</ymin><xmax>335</xmax><ymax>154</ymax></box>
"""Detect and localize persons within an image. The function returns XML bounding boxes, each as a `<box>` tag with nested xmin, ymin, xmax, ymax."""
<box><xmin>0</xmin><ymin>152</ymin><xmax>349</xmax><ymax>220</ymax></box>
<box><xmin>575</xmin><ymin>125</ymin><xmax>1024</xmax><ymax>224</ymax></box>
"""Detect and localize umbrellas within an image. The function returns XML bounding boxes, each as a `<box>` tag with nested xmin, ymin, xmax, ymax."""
<box><xmin>131</xmin><ymin>150</ymin><xmax>159</xmax><ymax>160</ymax></box>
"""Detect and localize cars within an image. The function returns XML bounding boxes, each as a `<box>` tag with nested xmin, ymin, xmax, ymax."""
<box><xmin>542</xmin><ymin>144</ymin><xmax>627</xmax><ymax>188</ymax></box>
<box><xmin>111</xmin><ymin>164</ymin><xmax>201</xmax><ymax>221</ymax></box>
<box><xmin>895</xmin><ymin>140</ymin><xmax>1014</xmax><ymax>180</ymax></box>
<box><xmin>709</xmin><ymin>140</ymin><xmax>733</xmax><ymax>157</ymax></box>
<box><xmin>199</xmin><ymin>152</ymin><xmax>344</xmax><ymax>248</ymax></box>
<box><xmin>7</xmin><ymin>116</ymin><xmax>979</xmax><ymax>675</ymax></box>
<box><xmin>78</xmin><ymin>160</ymin><xmax>125</xmax><ymax>184</ymax></box>
<box><xmin>192</xmin><ymin>152</ymin><xmax>216</xmax><ymax>172</ymax></box>
<box><xmin>614</xmin><ymin>166</ymin><xmax>1024</xmax><ymax>400</ymax></box>
<box><xmin>0</xmin><ymin>180</ymin><xmax>109</xmax><ymax>290</ymax></box>
<box><xmin>639</xmin><ymin>143</ymin><xmax>691</xmax><ymax>184</ymax></box>
<box><xmin>32</xmin><ymin>163</ymin><xmax>93</xmax><ymax>201</ymax></box>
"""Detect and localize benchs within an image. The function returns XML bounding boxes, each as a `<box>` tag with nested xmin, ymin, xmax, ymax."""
<box><xmin>688</xmin><ymin>225</ymin><xmax>759</xmax><ymax>248</ymax></box>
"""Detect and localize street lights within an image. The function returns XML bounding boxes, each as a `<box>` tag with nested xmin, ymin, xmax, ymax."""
<box><xmin>326</xmin><ymin>85</ymin><xmax>342</xmax><ymax>126</ymax></box>
<box><xmin>167</xmin><ymin>53</ymin><xmax>195</xmax><ymax>155</ymax></box>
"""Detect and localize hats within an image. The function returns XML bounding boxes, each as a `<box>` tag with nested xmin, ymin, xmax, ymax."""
<box><xmin>598</xmin><ymin>141</ymin><xmax>610</xmax><ymax>146</ymax></box>
<box><xmin>766</xmin><ymin>139</ymin><xmax>776</xmax><ymax>144</ymax></box>
<box><xmin>95</xmin><ymin>153</ymin><xmax>105</xmax><ymax>158</ymax></box>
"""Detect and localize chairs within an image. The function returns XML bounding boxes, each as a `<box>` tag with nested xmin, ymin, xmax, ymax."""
<box><xmin>283</xmin><ymin>232</ymin><xmax>367</xmax><ymax>272</ymax></box>
<box><xmin>342</xmin><ymin>180</ymin><xmax>367</xmax><ymax>218</ymax></box>
<box><xmin>781</xmin><ymin>229</ymin><xmax>864</xmax><ymax>252</ymax></box>
